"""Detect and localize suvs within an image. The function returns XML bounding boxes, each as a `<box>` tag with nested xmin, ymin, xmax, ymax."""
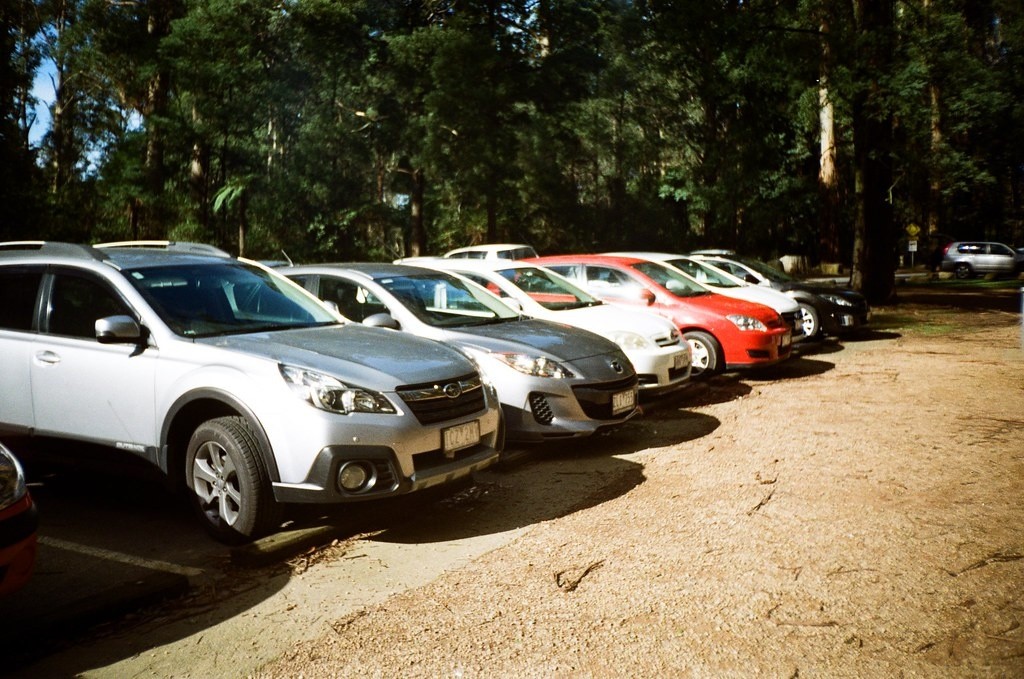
<box><xmin>1</xmin><ymin>234</ymin><xmax>506</xmax><ymax>548</ymax></box>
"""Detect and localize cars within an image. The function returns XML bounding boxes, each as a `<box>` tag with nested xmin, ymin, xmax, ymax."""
<box><xmin>388</xmin><ymin>256</ymin><xmax>694</xmax><ymax>400</ymax></box>
<box><xmin>938</xmin><ymin>240</ymin><xmax>1024</xmax><ymax>281</ymax></box>
<box><xmin>481</xmin><ymin>252</ymin><xmax>792</xmax><ymax>386</ymax></box>
<box><xmin>594</xmin><ymin>245</ymin><xmax>873</xmax><ymax>357</ymax></box>
<box><xmin>218</xmin><ymin>260</ymin><xmax>642</xmax><ymax>453</ymax></box>
<box><xmin>432</xmin><ymin>243</ymin><xmax>543</xmax><ymax>311</ymax></box>
<box><xmin>0</xmin><ymin>441</ymin><xmax>43</xmax><ymax>620</ymax></box>
